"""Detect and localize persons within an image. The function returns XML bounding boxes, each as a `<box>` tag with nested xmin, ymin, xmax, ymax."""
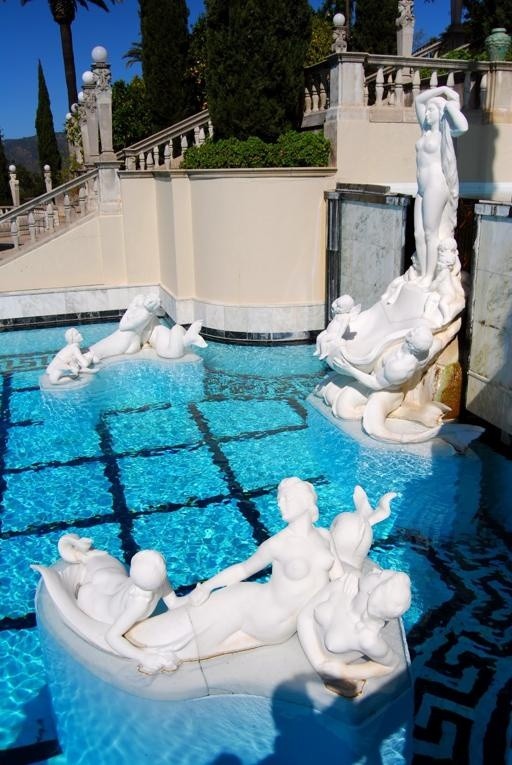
<box><xmin>312</xmin><ymin>87</ymin><xmax>470</xmax><ymax>390</ymax></box>
<box><xmin>78</xmin><ymin>478</ymin><xmax>410</xmax><ymax>679</ymax></box>
<box><xmin>45</xmin><ymin>327</ymin><xmax>99</xmax><ymax>383</ymax></box>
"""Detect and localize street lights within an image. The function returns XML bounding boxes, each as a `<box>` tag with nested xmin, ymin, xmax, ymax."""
<box><xmin>43</xmin><ymin>164</ymin><xmax>52</xmax><ymax>194</ymax></box>
<box><xmin>8</xmin><ymin>164</ymin><xmax>20</xmax><ymax>206</ymax></box>
<box><xmin>331</xmin><ymin>13</ymin><xmax>347</xmax><ymax>52</ymax></box>
<box><xmin>65</xmin><ymin>45</ymin><xmax>113</xmax><ymax>163</ymax></box>
